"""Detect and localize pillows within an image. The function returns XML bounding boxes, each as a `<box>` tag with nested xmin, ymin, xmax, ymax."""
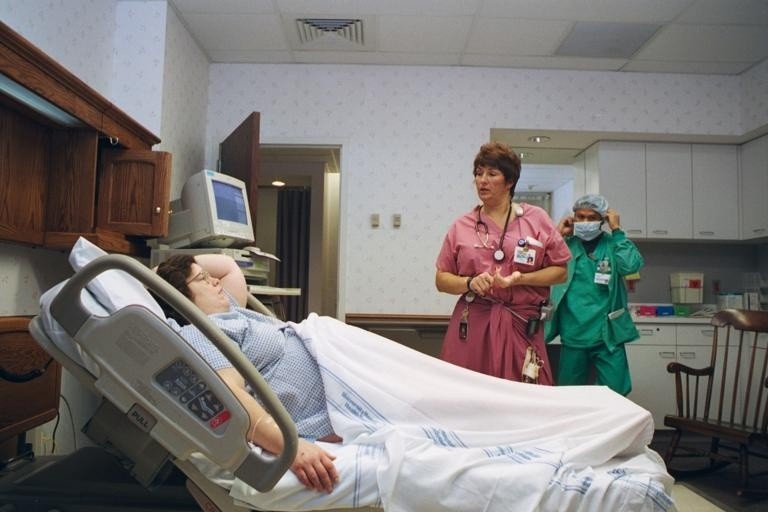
<box><xmin>68</xmin><ymin>237</ymin><xmax>167</xmax><ymax>329</ymax></box>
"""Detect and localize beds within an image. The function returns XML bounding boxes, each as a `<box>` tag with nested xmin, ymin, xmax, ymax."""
<box><xmin>28</xmin><ymin>254</ymin><xmax>670</xmax><ymax>512</ymax></box>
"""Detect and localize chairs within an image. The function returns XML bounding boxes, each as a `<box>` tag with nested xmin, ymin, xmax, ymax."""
<box><xmin>664</xmin><ymin>308</ymin><xmax>768</xmax><ymax>502</ymax></box>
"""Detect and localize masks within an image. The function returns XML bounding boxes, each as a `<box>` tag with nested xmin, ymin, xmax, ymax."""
<box><xmin>573</xmin><ymin>222</ymin><xmax>603</xmax><ymax>242</ymax></box>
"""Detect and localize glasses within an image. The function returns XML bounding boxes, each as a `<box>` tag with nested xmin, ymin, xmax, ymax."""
<box><xmin>186</xmin><ymin>271</ymin><xmax>208</xmax><ymax>286</ymax></box>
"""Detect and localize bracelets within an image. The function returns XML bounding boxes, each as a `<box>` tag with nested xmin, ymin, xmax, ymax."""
<box><xmin>466</xmin><ymin>271</ymin><xmax>477</xmax><ymax>291</ymax></box>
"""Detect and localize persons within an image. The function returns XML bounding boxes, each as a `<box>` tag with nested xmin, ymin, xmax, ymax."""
<box><xmin>542</xmin><ymin>192</ymin><xmax>643</xmax><ymax>398</ymax></box>
<box><xmin>432</xmin><ymin>141</ymin><xmax>571</xmax><ymax>385</ymax></box>
<box><xmin>146</xmin><ymin>251</ymin><xmax>346</xmax><ymax>497</ymax></box>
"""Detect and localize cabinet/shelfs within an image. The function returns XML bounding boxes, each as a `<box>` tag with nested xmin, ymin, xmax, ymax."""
<box><xmin>600</xmin><ymin>134</ymin><xmax>768</xmax><ymax>239</ymax></box>
<box><xmin>624</xmin><ymin>324</ymin><xmax>768</xmax><ymax>438</ymax></box>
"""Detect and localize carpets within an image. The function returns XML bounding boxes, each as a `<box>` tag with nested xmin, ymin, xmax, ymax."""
<box><xmin>669</xmin><ymin>481</ymin><xmax>739</xmax><ymax>512</ymax></box>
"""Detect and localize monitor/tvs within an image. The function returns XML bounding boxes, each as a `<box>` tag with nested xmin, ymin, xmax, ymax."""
<box><xmin>158</xmin><ymin>170</ymin><xmax>256</xmax><ymax>249</ymax></box>
